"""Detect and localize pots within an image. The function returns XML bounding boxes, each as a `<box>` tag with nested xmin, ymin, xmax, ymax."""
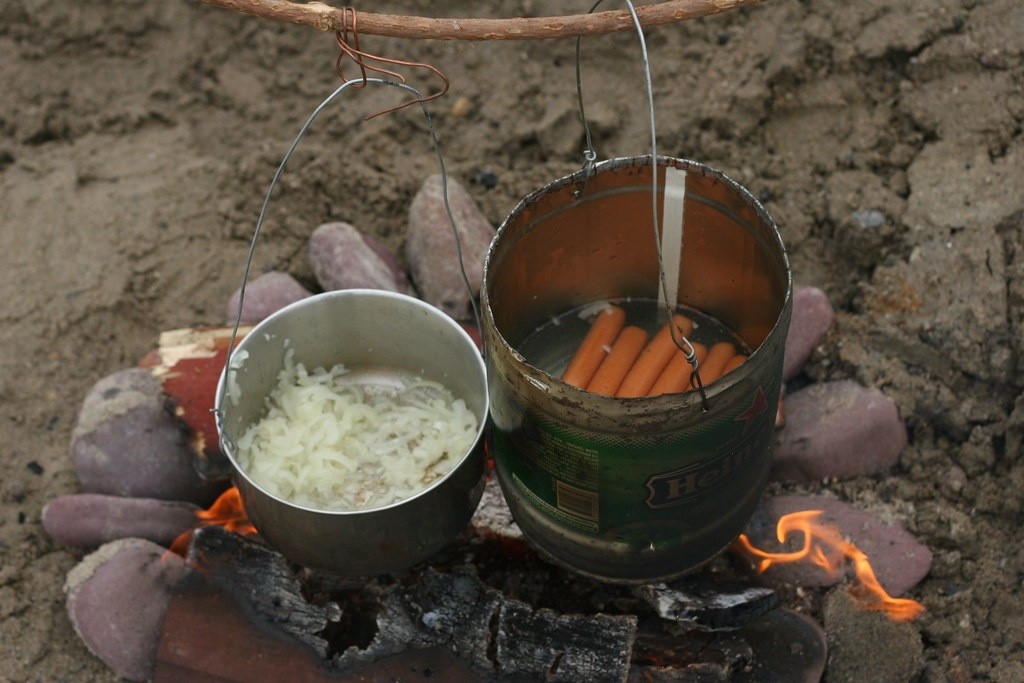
<box><xmin>213</xmin><ymin>285</ymin><xmax>490</xmax><ymax>586</ymax></box>
<box><xmin>479</xmin><ymin>154</ymin><xmax>795</xmax><ymax>591</ymax></box>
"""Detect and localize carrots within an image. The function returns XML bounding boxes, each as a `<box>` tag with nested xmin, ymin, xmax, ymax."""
<box><xmin>561</xmin><ymin>307</ymin><xmax>747</xmax><ymax>398</ymax></box>
